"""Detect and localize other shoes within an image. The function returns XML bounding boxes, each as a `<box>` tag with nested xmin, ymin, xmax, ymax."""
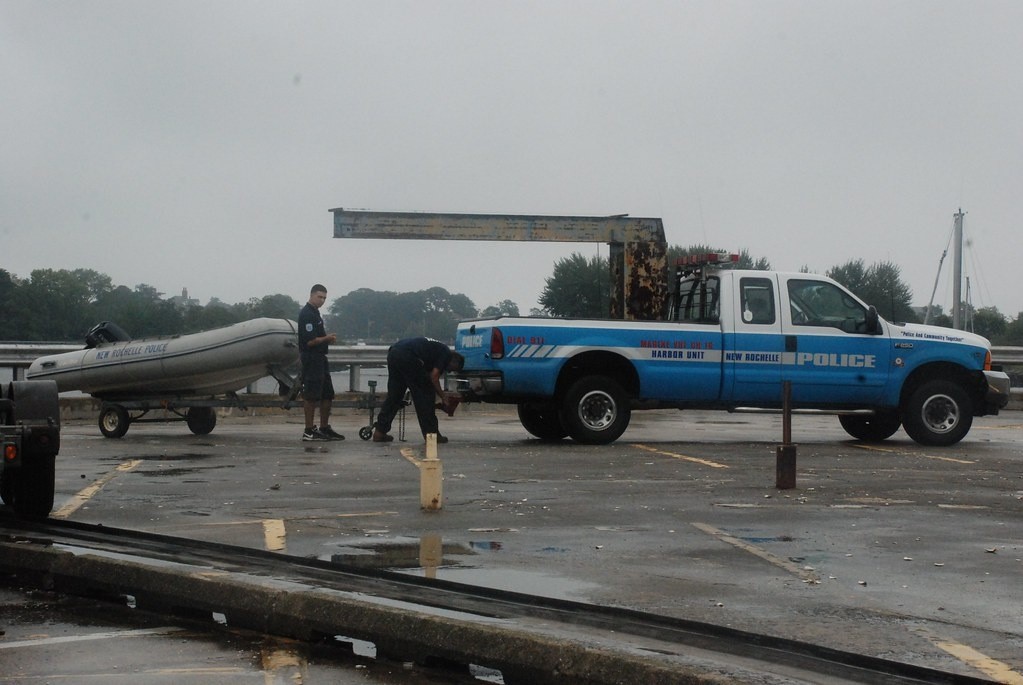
<box><xmin>373</xmin><ymin>431</ymin><xmax>394</xmax><ymax>442</ymax></box>
<box><xmin>438</xmin><ymin>433</ymin><xmax>448</xmax><ymax>443</ymax></box>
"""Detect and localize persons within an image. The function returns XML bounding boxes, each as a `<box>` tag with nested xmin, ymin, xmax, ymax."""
<box><xmin>298</xmin><ymin>284</ymin><xmax>345</xmax><ymax>441</ymax></box>
<box><xmin>373</xmin><ymin>337</ymin><xmax>465</xmax><ymax>443</ymax></box>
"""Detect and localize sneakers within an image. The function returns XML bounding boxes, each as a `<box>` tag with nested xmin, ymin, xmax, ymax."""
<box><xmin>302</xmin><ymin>425</ymin><xmax>329</xmax><ymax>440</ymax></box>
<box><xmin>319</xmin><ymin>425</ymin><xmax>345</xmax><ymax>440</ymax></box>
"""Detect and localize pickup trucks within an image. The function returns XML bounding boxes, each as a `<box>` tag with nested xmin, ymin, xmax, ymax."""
<box><xmin>0</xmin><ymin>381</ymin><xmax>62</xmax><ymax>517</ymax></box>
<box><xmin>453</xmin><ymin>270</ymin><xmax>1011</xmax><ymax>445</ymax></box>
